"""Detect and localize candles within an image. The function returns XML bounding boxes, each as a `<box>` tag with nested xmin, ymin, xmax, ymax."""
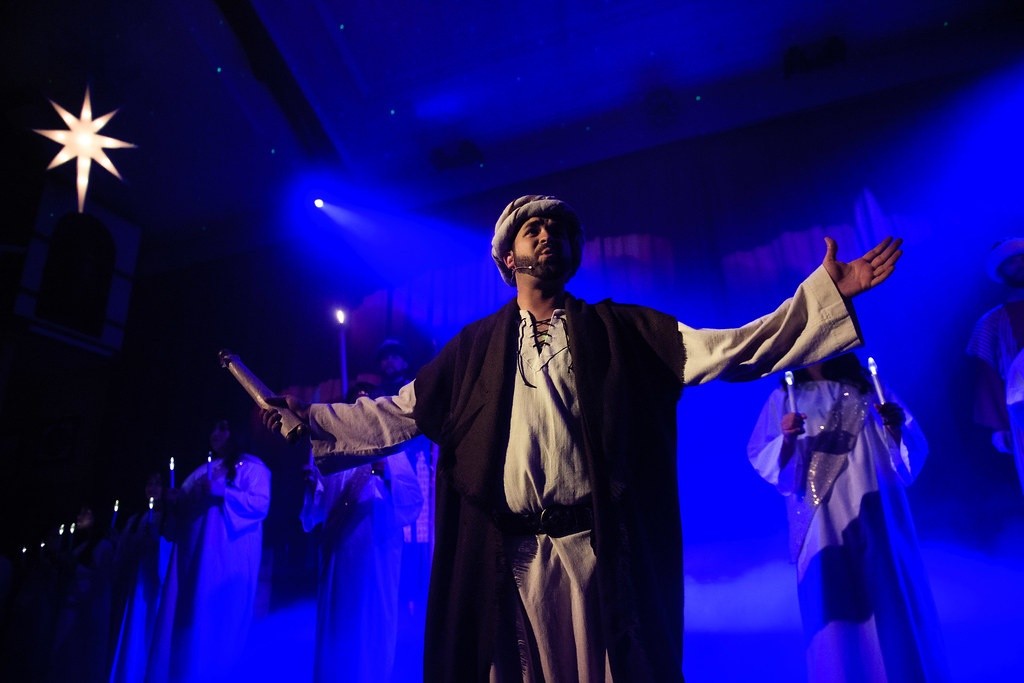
<box><xmin>208</xmin><ymin>456</ymin><xmax>212</xmax><ymax>481</ymax></box>
<box><xmin>149</xmin><ymin>502</ymin><xmax>154</xmax><ymax>523</ymax></box>
<box><xmin>788</xmin><ymin>384</ymin><xmax>797</xmax><ymax>413</ymax></box>
<box><xmin>338</xmin><ymin>323</ymin><xmax>349</xmax><ymax>397</ymax></box>
<box><xmin>871</xmin><ymin>374</ymin><xmax>886</xmax><ymax>405</ymax></box>
<box><xmin>169</xmin><ymin>463</ymin><xmax>175</xmax><ymax>488</ymax></box>
<box><xmin>112</xmin><ymin>505</ymin><xmax>118</xmax><ymax>528</ymax></box>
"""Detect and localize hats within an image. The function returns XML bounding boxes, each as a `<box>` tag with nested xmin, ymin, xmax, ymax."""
<box><xmin>375</xmin><ymin>338</ymin><xmax>411</xmax><ymax>366</ymax></box>
<box><xmin>490</xmin><ymin>194</ymin><xmax>584</xmax><ymax>288</ymax></box>
<box><xmin>984</xmin><ymin>237</ymin><xmax>1024</xmax><ymax>292</ymax></box>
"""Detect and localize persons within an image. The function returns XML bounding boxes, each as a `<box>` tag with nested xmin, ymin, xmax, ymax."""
<box><xmin>256</xmin><ymin>193</ymin><xmax>906</xmax><ymax>680</ymax></box>
<box><xmin>2</xmin><ymin>189</ymin><xmax>437</xmax><ymax>683</ymax></box>
<box><xmin>964</xmin><ymin>236</ymin><xmax>1024</xmax><ymax>487</ymax></box>
<box><xmin>748</xmin><ymin>350</ymin><xmax>935</xmax><ymax>681</ymax></box>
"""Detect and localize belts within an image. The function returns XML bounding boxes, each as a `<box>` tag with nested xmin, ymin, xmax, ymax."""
<box><xmin>502</xmin><ymin>488</ymin><xmax>596</xmax><ymax>539</ymax></box>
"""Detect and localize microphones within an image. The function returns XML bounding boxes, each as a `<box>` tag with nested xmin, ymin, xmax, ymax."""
<box><xmin>512</xmin><ymin>266</ymin><xmax>531</xmax><ymax>278</ymax></box>
